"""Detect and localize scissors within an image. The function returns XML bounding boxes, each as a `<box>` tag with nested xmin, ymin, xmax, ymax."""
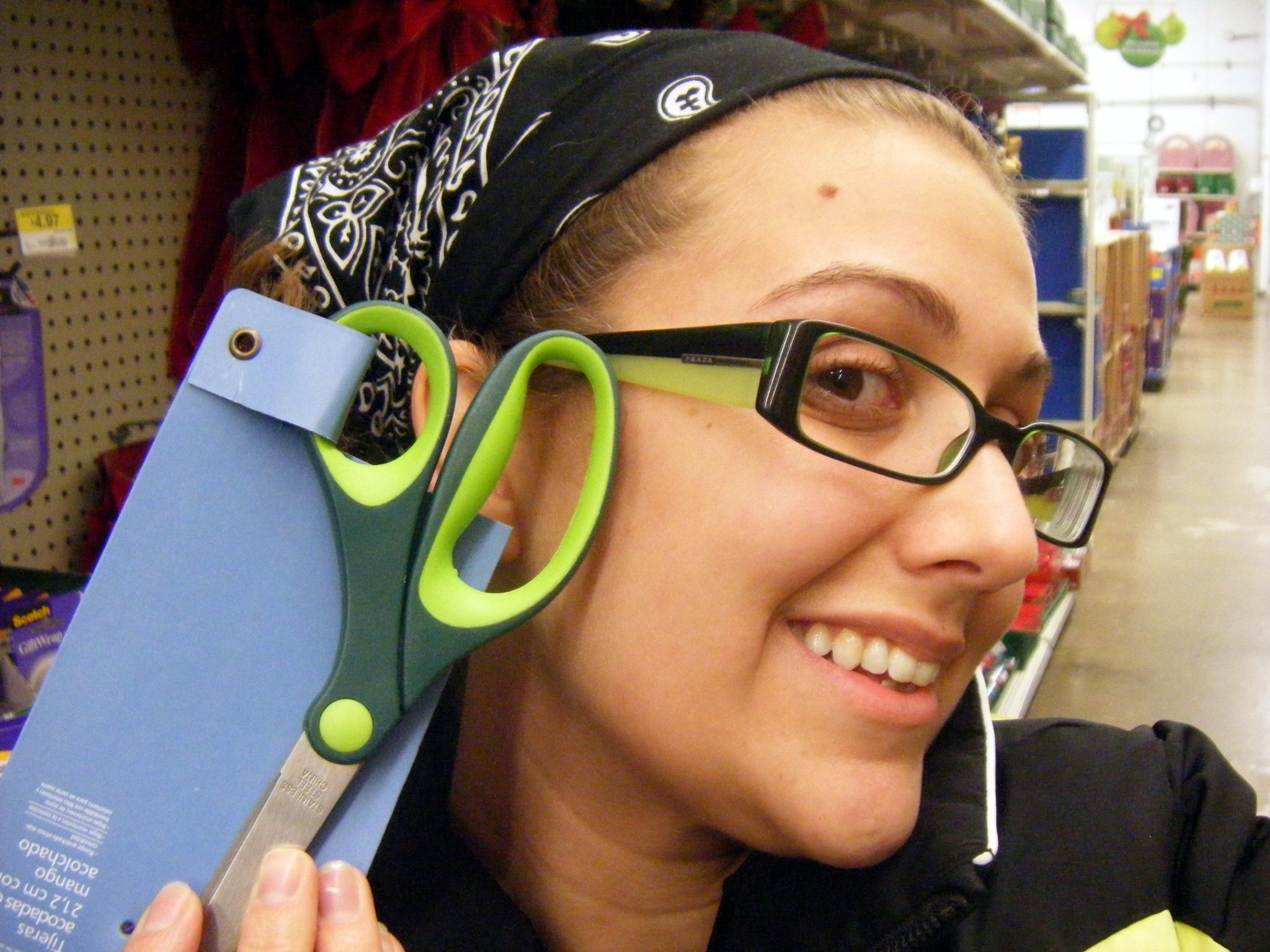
<box><xmin>198</xmin><ymin>299</ymin><xmax>620</xmax><ymax>952</ymax></box>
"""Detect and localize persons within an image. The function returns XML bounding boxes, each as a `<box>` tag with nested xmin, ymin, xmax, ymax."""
<box><xmin>126</xmin><ymin>30</ymin><xmax>1270</xmax><ymax>952</ymax></box>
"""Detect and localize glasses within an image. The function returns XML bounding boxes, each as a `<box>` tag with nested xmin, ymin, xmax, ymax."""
<box><xmin>544</xmin><ymin>319</ymin><xmax>1117</xmax><ymax>550</ymax></box>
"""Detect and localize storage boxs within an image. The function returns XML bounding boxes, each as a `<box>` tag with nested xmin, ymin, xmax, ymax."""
<box><xmin>1201</xmin><ymin>245</ymin><xmax>1254</xmax><ymax>320</ymax></box>
<box><xmin>1093</xmin><ymin>230</ymin><xmax>1179</xmax><ymax>456</ymax></box>
